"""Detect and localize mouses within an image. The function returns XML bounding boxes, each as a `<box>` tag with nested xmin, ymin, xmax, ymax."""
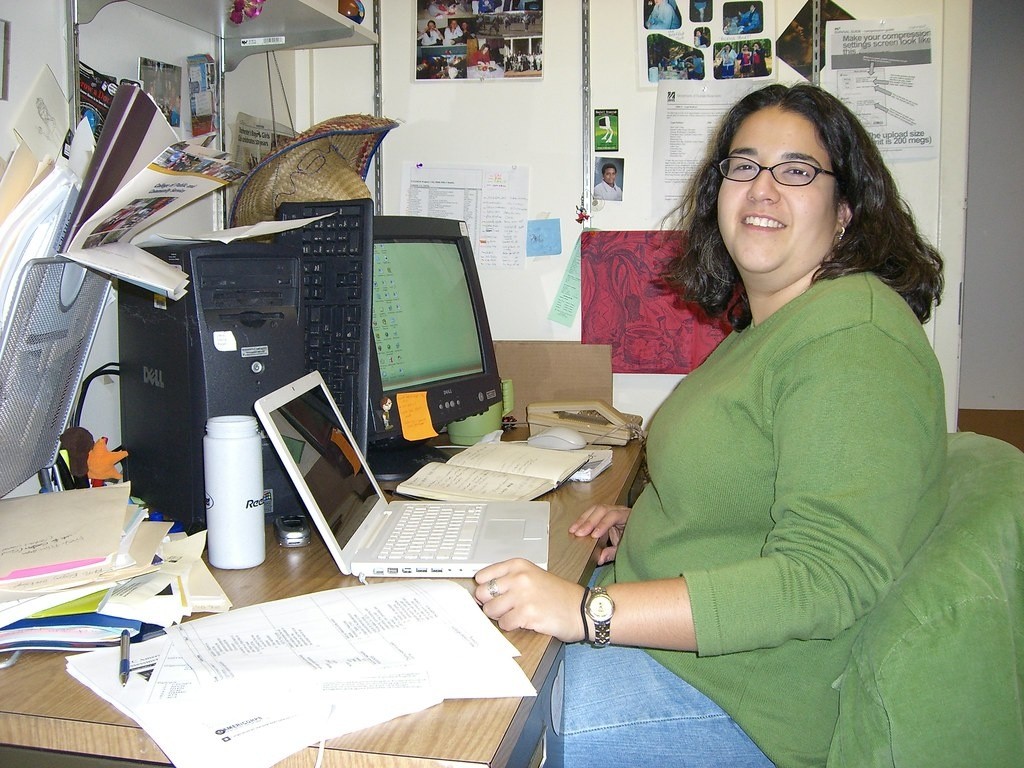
<box><xmin>525</xmin><ymin>426</ymin><xmax>586</xmax><ymax>451</ymax></box>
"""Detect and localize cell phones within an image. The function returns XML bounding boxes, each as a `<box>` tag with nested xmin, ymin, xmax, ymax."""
<box><xmin>273</xmin><ymin>514</ymin><xmax>309</xmax><ymax>548</ymax></box>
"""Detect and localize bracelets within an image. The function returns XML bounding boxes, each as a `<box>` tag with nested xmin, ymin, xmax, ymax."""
<box><xmin>581</xmin><ymin>586</ymin><xmax>589</xmax><ymax>644</ymax></box>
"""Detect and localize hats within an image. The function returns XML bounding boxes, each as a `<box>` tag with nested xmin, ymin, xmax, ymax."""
<box><xmin>226</xmin><ymin>114</ymin><xmax>401</xmax><ymax>239</ymax></box>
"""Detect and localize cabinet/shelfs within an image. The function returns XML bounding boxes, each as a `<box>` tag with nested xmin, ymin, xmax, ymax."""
<box><xmin>66</xmin><ymin>0</ymin><xmax>382</xmax><ymax>240</ymax></box>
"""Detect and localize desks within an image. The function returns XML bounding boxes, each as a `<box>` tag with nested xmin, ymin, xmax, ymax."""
<box><xmin>0</xmin><ymin>414</ymin><xmax>647</xmax><ymax>768</ymax></box>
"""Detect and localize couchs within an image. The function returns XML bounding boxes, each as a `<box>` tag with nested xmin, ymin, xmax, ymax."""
<box><xmin>824</xmin><ymin>432</ymin><xmax>1024</xmax><ymax>768</ymax></box>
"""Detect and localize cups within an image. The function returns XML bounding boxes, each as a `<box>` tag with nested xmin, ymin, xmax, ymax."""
<box><xmin>447</xmin><ymin>378</ymin><xmax>515</xmax><ymax>445</ymax></box>
<box><xmin>202</xmin><ymin>415</ymin><xmax>266</xmax><ymax>569</ymax></box>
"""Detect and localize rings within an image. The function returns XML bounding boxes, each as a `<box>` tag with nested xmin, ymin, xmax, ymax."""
<box><xmin>488</xmin><ymin>578</ymin><xmax>501</xmax><ymax>598</ymax></box>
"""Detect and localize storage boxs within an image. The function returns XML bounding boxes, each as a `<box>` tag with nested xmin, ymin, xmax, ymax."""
<box><xmin>495</xmin><ymin>339</ymin><xmax>613</xmax><ymax>409</ymax></box>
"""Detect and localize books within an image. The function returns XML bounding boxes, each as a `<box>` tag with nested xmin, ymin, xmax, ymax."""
<box><xmin>396</xmin><ymin>441</ymin><xmax>612</xmax><ymax>502</ymax></box>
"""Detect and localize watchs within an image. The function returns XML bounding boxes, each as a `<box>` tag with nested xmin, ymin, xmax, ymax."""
<box><xmin>585</xmin><ymin>584</ymin><xmax>615</xmax><ymax>648</ymax></box>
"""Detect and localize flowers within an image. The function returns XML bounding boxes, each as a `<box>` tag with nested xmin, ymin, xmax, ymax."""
<box><xmin>225</xmin><ymin>0</ymin><xmax>265</xmax><ymax>25</ymax></box>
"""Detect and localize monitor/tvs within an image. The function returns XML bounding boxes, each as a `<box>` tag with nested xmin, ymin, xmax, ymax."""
<box><xmin>365</xmin><ymin>215</ymin><xmax>503</xmax><ymax>481</ymax></box>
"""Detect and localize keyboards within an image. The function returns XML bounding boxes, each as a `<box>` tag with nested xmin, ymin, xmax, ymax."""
<box><xmin>277</xmin><ymin>196</ymin><xmax>374</xmax><ymax>474</ymax></box>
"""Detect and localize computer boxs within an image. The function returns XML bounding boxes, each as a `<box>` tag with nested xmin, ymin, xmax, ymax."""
<box><xmin>120</xmin><ymin>235</ymin><xmax>309</xmax><ymax>542</ymax></box>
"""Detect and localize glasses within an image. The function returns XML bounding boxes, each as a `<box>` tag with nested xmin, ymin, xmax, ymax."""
<box><xmin>710</xmin><ymin>156</ymin><xmax>840</xmax><ymax>187</ymax></box>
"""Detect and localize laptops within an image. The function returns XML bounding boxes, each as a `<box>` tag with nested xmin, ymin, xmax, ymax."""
<box><xmin>253</xmin><ymin>370</ymin><xmax>550</xmax><ymax>577</ymax></box>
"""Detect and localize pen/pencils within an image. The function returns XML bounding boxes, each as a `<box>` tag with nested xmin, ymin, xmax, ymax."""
<box><xmin>118</xmin><ymin>629</ymin><xmax>131</xmax><ymax>688</ymax></box>
<box><xmin>38</xmin><ymin>451</ymin><xmax>75</xmax><ymax>493</ymax></box>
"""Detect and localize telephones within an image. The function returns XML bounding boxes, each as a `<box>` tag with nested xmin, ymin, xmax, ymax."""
<box><xmin>524</xmin><ymin>400</ymin><xmax>644</xmax><ymax>447</ymax></box>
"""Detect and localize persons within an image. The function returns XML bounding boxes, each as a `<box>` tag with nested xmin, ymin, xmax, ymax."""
<box><xmin>414</xmin><ymin>0</ymin><xmax>815</xmax><ymax>80</ymax></box>
<box><xmin>474</xmin><ymin>80</ymin><xmax>947</xmax><ymax>768</ymax></box>
<box><xmin>593</xmin><ymin>163</ymin><xmax>623</xmax><ymax>200</ymax></box>
